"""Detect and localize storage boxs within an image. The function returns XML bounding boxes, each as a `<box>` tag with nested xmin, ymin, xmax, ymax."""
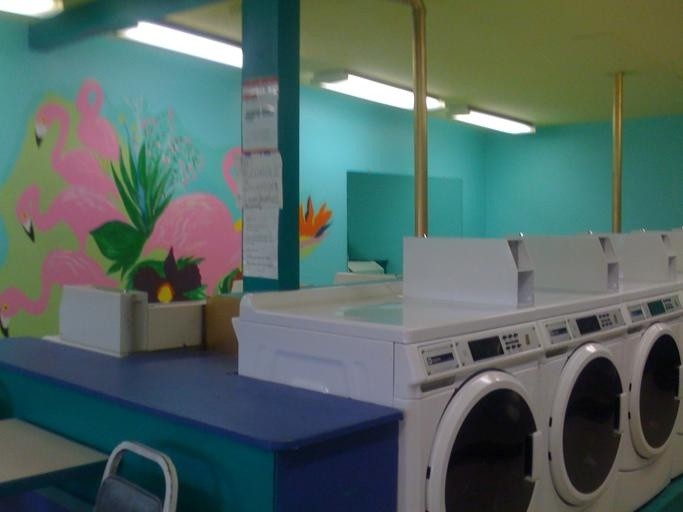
<box><xmin>133</xmin><ymin>291</ymin><xmax>208</xmax><ymax>354</ymax></box>
<box><xmin>204</xmin><ymin>297</ymin><xmax>243</xmax><ymax>356</ymax></box>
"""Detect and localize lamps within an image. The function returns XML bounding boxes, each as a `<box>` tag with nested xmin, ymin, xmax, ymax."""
<box><xmin>309</xmin><ymin>69</ymin><xmax>447</xmax><ymax>115</ymax></box>
<box><xmin>114</xmin><ymin>17</ymin><xmax>243</xmax><ymax>71</ymax></box>
<box><xmin>1</xmin><ymin>0</ymin><xmax>65</xmax><ymax>20</ymax></box>
<box><xmin>452</xmin><ymin>105</ymin><xmax>536</xmax><ymax>136</ymax></box>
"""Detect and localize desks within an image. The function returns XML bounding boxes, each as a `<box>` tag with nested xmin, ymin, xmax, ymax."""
<box><xmin>0</xmin><ymin>417</ymin><xmax>110</xmax><ymax>496</ymax></box>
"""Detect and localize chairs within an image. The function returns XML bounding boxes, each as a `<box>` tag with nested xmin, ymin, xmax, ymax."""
<box><xmin>89</xmin><ymin>441</ymin><xmax>179</xmax><ymax>512</ymax></box>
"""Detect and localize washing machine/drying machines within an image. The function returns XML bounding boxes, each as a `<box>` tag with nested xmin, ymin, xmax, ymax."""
<box><xmin>236</xmin><ymin>284</ymin><xmax>551</xmax><ymax>512</ymax></box>
<box><xmin>353</xmin><ymin>278</ymin><xmax>632</xmax><ymax>511</ymax></box>
<box><xmin>521</xmin><ymin>273</ymin><xmax>683</xmax><ymax>512</ymax></box>
